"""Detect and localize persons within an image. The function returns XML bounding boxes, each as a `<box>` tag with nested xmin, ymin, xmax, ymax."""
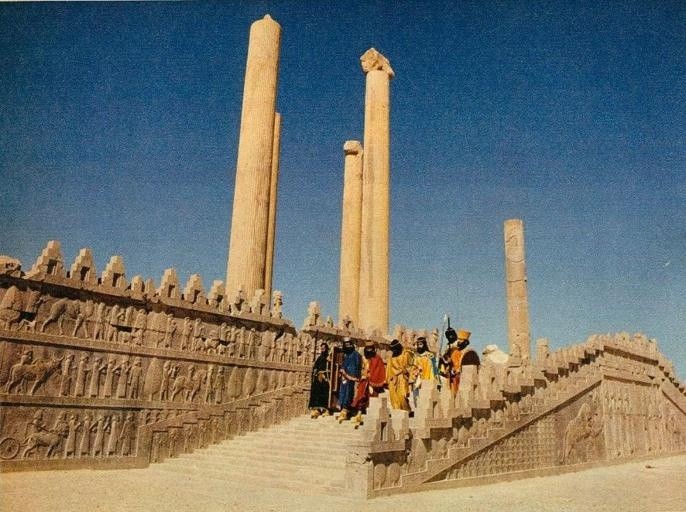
<box><xmin>308</xmin><ymin>342</ymin><xmax>330</xmax><ymax>420</ymax></box>
<box><xmin>439</xmin><ymin>326</ymin><xmax>460</xmax><ymax>361</ymax></box>
<box><xmin>0</xmin><ymin>286</ymin><xmax>311</xmax><ymax>458</ymax></box>
<box><xmin>409</xmin><ymin>336</ymin><xmax>442</xmax><ymax>408</ymax></box>
<box><xmin>350</xmin><ymin>341</ymin><xmax>386</xmax><ymax>424</ymax></box>
<box><xmin>438</xmin><ymin>330</ymin><xmax>481</xmax><ymax>395</ymax></box>
<box><xmin>385</xmin><ymin>339</ymin><xmax>413</xmax><ymax>418</ymax></box>
<box><xmin>332</xmin><ymin>336</ymin><xmax>362</xmax><ymax>422</ymax></box>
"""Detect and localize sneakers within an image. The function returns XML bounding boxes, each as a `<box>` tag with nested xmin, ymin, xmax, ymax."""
<box><xmin>311</xmin><ymin>408</ymin><xmax>330</xmax><ymax>418</ymax></box>
<box><xmin>338</xmin><ymin>409</ymin><xmax>363</xmax><ymax>428</ymax></box>
